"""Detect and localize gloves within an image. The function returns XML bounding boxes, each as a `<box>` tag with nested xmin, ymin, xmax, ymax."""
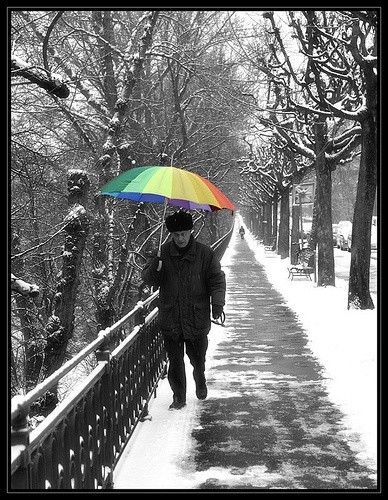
<box><xmin>151</xmin><ymin>256</ymin><xmax>162</xmax><ymax>270</ymax></box>
<box><xmin>212</xmin><ymin>304</ymin><xmax>223</xmax><ymax>319</ymax></box>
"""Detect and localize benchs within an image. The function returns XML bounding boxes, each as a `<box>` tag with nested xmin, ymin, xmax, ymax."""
<box><xmin>288</xmin><ymin>250</ymin><xmax>313</xmax><ymax>281</ymax></box>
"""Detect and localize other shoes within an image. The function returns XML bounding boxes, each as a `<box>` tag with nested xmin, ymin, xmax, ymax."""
<box><xmin>193</xmin><ymin>369</ymin><xmax>207</xmax><ymax>399</ymax></box>
<box><xmin>169</xmin><ymin>401</ymin><xmax>186</xmax><ymax>410</ymax></box>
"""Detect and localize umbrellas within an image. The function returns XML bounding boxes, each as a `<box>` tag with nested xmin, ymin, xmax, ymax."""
<box><xmin>96</xmin><ymin>154</ymin><xmax>235</xmax><ymax>271</ymax></box>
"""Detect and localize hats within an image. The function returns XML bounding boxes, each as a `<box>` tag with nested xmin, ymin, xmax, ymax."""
<box><xmin>165</xmin><ymin>210</ymin><xmax>193</xmax><ymax>232</ymax></box>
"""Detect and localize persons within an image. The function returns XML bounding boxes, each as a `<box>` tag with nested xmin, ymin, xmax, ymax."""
<box><xmin>142</xmin><ymin>212</ymin><xmax>226</xmax><ymax>409</ymax></box>
<box><xmin>239</xmin><ymin>226</ymin><xmax>244</xmax><ymax>239</ymax></box>
